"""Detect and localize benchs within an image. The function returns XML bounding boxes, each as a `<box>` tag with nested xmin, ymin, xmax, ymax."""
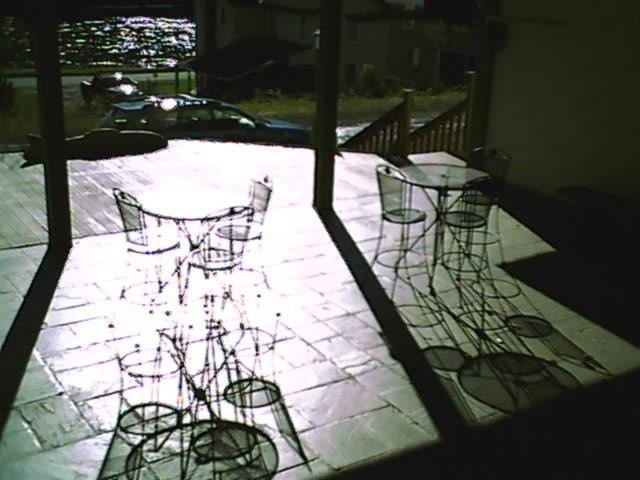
<box><xmin>558</xmin><ymin>186</ymin><xmax>640</xmax><ymax>226</ymax></box>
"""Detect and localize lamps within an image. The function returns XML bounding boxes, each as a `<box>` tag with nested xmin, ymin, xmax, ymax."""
<box><xmin>422</xmin><ymin>17</ymin><xmax>477</xmax><ymax>42</ymax></box>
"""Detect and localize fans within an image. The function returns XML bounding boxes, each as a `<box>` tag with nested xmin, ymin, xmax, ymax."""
<box><xmin>347</xmin><ymin>0</ymin><xmax>565</xmax><ymax>27</ymax></box>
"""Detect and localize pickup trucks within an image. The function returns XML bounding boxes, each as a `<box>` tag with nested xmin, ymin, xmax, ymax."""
<box><xmin>80</xmin><ymin>72</ymin><xmax>144</xmax><ymax>116</ymax></box>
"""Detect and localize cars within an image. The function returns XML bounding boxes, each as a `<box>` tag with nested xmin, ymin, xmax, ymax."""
<box><xmin>96</xmin><ymin>94</ymin><xmax>311</xmax><ymax>149</ymax></box>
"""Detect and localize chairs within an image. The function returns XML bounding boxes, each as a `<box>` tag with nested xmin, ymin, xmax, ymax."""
<box><xmin>359</xmin><ymin>145</ymin><xmax>513</xmax><ymax>305</ymax></box>
<box><xmin>107</xmin><ymin>169</ymin><xmax>282</xmax><ymax>350</ymax></box>
